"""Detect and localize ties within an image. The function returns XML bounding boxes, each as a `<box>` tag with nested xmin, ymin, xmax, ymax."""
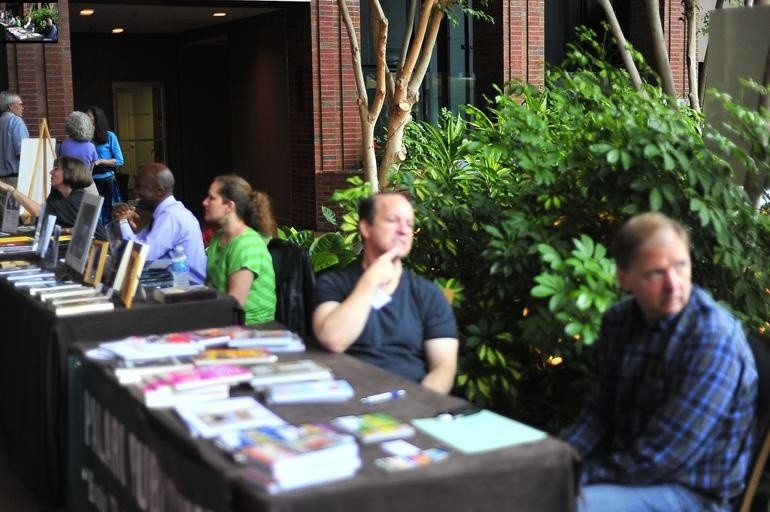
<box><xmin>148</xmin><ymin>218</ymin><xmax>153</xmax><ymax>232</ymax></box>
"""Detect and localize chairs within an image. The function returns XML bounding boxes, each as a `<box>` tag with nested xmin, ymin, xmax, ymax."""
<box><xmin>264</xmin><ymin>237</ymin><xmax>321</xmax><ymax>348</ymax></box>
<box><xmin>740</xmin><ymin>333</ymin><xmax>770</xmax><ymax>512</ymax></box>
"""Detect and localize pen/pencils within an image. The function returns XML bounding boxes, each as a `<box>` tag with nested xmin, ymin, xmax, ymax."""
<box><xmin>360</xmin><ymin>390</ymin><xmax>406</xmax><ymax>404</ymax></box>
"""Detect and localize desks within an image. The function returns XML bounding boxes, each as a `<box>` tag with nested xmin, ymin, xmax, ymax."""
<box><xmin>0</xmin><ymin>257</ymin><xmax>248</xmax><ymax>508</ymax></box>
<box><xmin>67</xmin><ymin>319</ymin><xmax>581</xmax><ymax>510</ymax></box>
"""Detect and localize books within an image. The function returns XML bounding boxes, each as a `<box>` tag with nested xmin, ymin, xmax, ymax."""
<box><xmin>0</xmin><ymin>190</ymin><xmax>217</xmax><ymax>321</ymax></box>
<box><xmin>95</xmin><ymin>316</ymin><xmax>548</xmax><ymax>497</ymax></box>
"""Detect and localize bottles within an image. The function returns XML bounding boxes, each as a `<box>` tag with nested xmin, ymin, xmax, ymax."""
<box><xmin>172</xmin><ymin>246</ymin><xmax>191</xmax><ymax>288</ymax></box>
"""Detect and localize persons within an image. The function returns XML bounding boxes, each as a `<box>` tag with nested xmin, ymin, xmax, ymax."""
<box><xmin>306</xmin><ymin>185</ymin><xmax>459</xmax><ymax>397</ymax></box>
<box><xmin>84</xmin><ymin>105</ymin><xmax>126</xmax><ymax>228</ymax></box>
<box><xmin>57</xmin><ymin>111</ymin><xmax>99</xmax><ymax>185</ymax></box>
<box><xmin>111</xmin><ymin>162</ymin><xmax>208</xmax><ymax>289</ymax></box>
<box><xmin>0</xmin><ymin>156</ymin><xmax>107</xmax><ymax>243</ymax></box>
<box><xmin>1</xmin><ymin>91</ymin><xmax>32</xmax><ymax>219</ymax></box>
<box><xmin>200</xmin><ymin>173</ymin><xmax>280</xmax><ymax>325</ymax></box>
<box><xmin>0</xmin><ymin>8</ymin><xmax>58</xmax><ymax>41</ymax></box>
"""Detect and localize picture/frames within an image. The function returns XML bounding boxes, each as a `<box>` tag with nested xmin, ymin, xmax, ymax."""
<box><xmin>64</xmin><ymin>193</ymin><xmax>147</xmax><ymax>307</ymax></box>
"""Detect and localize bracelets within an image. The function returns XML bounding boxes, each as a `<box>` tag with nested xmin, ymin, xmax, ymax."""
<box><xmin>9</xmin><ymin>187</ymin><xmax>17</xmax><ymax>194</ymax></box>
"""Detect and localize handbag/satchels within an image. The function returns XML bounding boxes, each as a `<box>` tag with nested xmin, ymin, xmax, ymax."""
<box><xmin>0</xmin><ymin>190</ymin><xmax>21</xmax><ymax>235</ymax></box>
